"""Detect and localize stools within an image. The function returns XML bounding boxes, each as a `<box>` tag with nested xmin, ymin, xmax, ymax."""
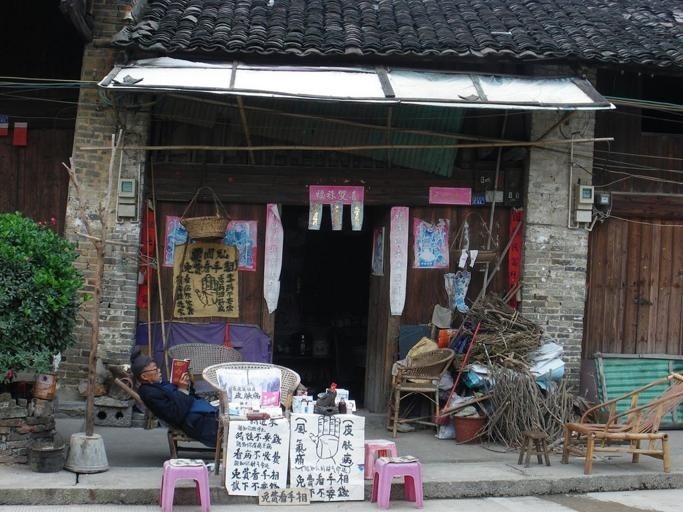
<box><xmin>365</xmin><ymin>438</ymin><xmax>398</xmax><ymax>481</ymax></box>
<box><xmin>160</xmin><ymin>459</ymin><xmax>212</xmax><ymax>512</ymax></box>
<box><xmin>371</xmin><ymin>456</ymin><xmax>424</xmax><ymax>508</ymax></box>
<box><xmin>518</xmin><ymin>431</ymin><xmax>551</xmax><ymax>468</ymax></box>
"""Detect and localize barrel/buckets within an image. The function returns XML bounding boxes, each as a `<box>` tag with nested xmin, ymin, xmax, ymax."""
<box><xmin>32</xmin><ymin>371</ymin><xmax>58</xmax><ymax>401</ymax></box>
<box><xmin>23</xmin><ymin>431</ymin><xmax>66</xmax><ymax>473</ymax></box>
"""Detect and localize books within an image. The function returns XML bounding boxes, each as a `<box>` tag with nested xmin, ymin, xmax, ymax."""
<box><xmin>381</xmin><ymin>454</ymin><xmax>418</xmax><ymax>463</ymax></box>
<box><xmin>167</xmin><ymin>457</ymin><xmax>203</xmax><ymax>467</ymax></box>
<box><xmin>169</xmin><ymin>358</ymin><xmax>191</xmax><ymax>385</ymax></box>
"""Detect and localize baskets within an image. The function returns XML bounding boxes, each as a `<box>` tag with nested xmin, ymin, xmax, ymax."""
<box><xmin>178</xmin><ymin>185</ymin><xmax>232</xmax><ymax>241</ymax></box>
<box><xmin>450</xmin><ymin>245</ymin><xmax>498</xmax><ymax>265</ymax></box>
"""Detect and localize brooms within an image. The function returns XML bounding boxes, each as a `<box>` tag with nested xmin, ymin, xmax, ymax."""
<box><xmin>431</xmin><ymin>322</ymin><xmax>480</xmax><ymax>425</ymax></box>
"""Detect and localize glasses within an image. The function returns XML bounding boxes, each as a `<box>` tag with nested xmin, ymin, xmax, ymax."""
<box><xmin>136</xmin><ymin>366</ymin><xmax>159</xmax><ymax>377</ymax></box>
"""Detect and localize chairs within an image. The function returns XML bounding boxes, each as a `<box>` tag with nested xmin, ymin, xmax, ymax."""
<box><xmin>202</xmin><ymin>362</ymin><xmax>302</xmax><ymax>487</ymax></box>
<box><xmin>168</xmin><ymin>343</ymin><xmax>242</xmax><ymax>405</ymax></box>
<box><xmin>561</xmin><ymin>371</ymin><xmax>683</xmax><ymax>475</ymax></box>
<box><xmin>113</xmin><ymin>376</ymin><xmax>223</xmax><ymax>460</ymax></box>
<box><xmin>386</xmin><ymin>348</ymin><xmax>456</xmax><ymax>438</ymax></box>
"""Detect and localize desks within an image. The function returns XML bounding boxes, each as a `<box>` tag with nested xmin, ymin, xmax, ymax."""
<box><xmin>278</xmin><ymin>353</ymin><xmax>312</xmax><ymax>360</ymax></box>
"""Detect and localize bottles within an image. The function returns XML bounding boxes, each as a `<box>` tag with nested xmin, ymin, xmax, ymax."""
<box><xmin>338</xmin><ymin>397</ymin><xmax>346</xmax><ymax>414</ymax></box>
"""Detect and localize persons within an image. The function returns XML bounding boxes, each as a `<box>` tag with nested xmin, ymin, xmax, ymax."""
<box><xmin>308</xmin><ymin>416</ymin><xmax>341</xmax><ymax>465</ymax></box>
<box><xmin>167</xmin><ymin>222</ymin><xmax>191</xmax><ymax>263</ymax></box>
<box><xmin>415</xmin><ymin>220</ymin><xmax>444</xmax><ymax>266</ymax></box>
<box><xmin>225</xmin><ymin>223</ymin><xmax>255</xmax><ymax>268</ymax></box>
<box><xmin>130</xmin><ymin>354</ymin><xmax>222</xmax><ymax>447</ymax></box>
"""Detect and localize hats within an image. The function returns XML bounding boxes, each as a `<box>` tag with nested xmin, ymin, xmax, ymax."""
<box><xmin>129</xmin><ymin>344</ymin><xmax>157</xmax><ymax>377</ymax></box>
